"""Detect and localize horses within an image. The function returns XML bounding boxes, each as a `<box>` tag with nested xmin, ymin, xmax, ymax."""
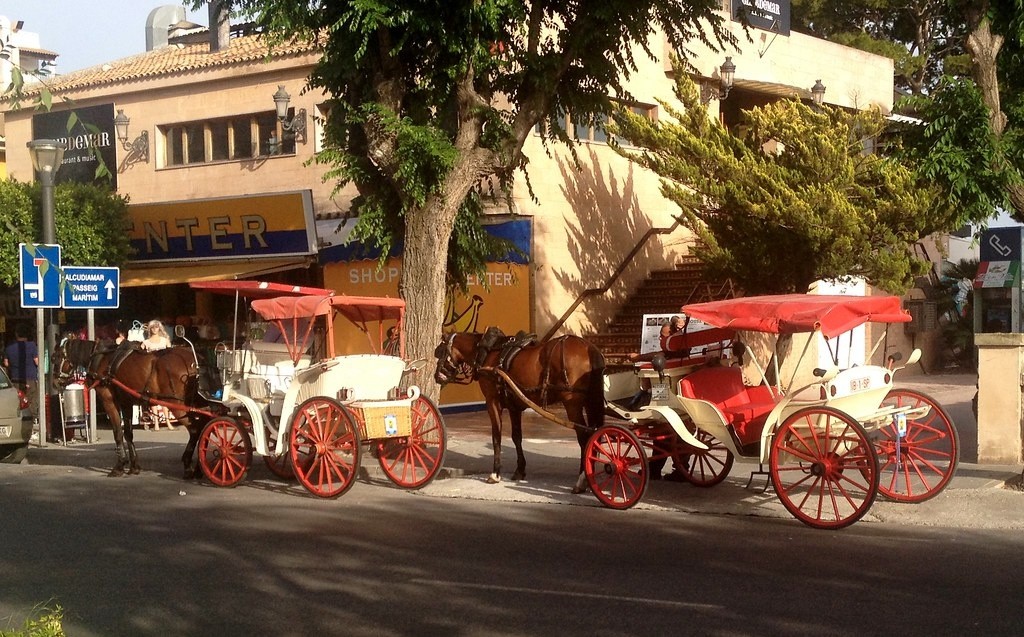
<box><xmin>50</xmin><ymin>333</ymin><xmax>214</xmax><ymax>478</ymax></box>
<box><xmin>433</xmin><ymin>322</ymin><xmax>606</xmax><ymax>495</ymax></box>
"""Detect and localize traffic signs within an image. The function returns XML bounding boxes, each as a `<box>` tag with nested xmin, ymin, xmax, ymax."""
<box><xmin>19</xmin><ymin>243</ymin><xmax>62</xmax><ymax>309</ymax></box>
<box><xmin>61</xmin><ymin>266</ymin><xmax>120</xmax><ymax>309</ymax></box>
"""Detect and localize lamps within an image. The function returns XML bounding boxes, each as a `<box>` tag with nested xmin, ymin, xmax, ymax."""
<box><xmin>811</xmin><ymin>79</ymin><xmax>826</xmax><ymax>105</ymax></box>
<box><xmin>700</xmin><ymin>56</ymin><xmax>737</xmax><ymax>106</ymax></box>
<box><xmin>271</xmin><ymin>85</ymin><xmax>307</xmax><ymax>145</ymax></box>
<box><xmin>112</xmin><ymin>109</ymin><xmax>150</xmax><ymax>163</ymax></box>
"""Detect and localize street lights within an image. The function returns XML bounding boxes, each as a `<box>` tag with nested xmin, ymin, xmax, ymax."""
<box><xmin>26</xmin><ymin>138</ymin><xmax>66</xmax><ymax>326</ymax></box>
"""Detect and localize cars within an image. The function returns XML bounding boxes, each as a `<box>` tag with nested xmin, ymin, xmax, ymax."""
<box><xmin>0</xmin><ymin>366</ymin><xmax>34</xmax><ymax>464</ymax></box>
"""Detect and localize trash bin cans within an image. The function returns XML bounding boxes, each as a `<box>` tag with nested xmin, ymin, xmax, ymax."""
<box><xmin>62</xmin><ymin>389</ymin><xmax>86</xmax><ymax>428</ymax></box>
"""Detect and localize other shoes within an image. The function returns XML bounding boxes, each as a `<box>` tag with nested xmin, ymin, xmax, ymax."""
<box><xmin>663</xmin><ymin>469</ymin><xmax>689</xmax><ymax>482</ymax></box>
<box><xmin>637</xmin><ymin>469</ymin><xmax>663</xmax><ymax>480</ymax></box>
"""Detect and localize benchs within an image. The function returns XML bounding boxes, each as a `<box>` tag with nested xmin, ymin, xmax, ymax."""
<box><xmin>775</xmin><ymin>365</ymin><xmax>893</xmax><ymax>429</ymax></box>
<box><xmin>216</xmin><ymin>341</ymin><xmax>312</xmax><ymax>376</ymax></box>
<box><xmin>294</xmin><ymin>355</ymin><xmax>406</xmax><ymax>408</ymax></box>
<box><xmin>246</xmin><ymin>376</ymin><xmax>293</xmax><ymax>416</ymax></box>
<box><xmin>677</xmin><ymin>365</ymin><xmax>786</xmax><ymax>427</ymax></box>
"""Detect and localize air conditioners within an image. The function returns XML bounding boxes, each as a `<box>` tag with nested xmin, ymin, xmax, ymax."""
<box><xmin>907</xmin><ymin>304</ymin><xmax>935</xmax><ymax>329</ymax></box>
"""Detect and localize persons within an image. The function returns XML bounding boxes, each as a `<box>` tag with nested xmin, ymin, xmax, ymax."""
<box><xmin>6</xmin><ymin>324</ymin><xmax>39</xmax><ymax>417</ymax></box>
<box><xmin>115</xmin><ymin>323</ymin><xmax>126</xmax><ymax>345</ymax></box>
<box><xmin>141</xmin><ymin>320</ymin><xmax>174</xmax><ymax>430</ymax></box>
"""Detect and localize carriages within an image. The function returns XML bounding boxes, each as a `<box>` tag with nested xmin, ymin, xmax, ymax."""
<box><xmin>50</xmin><ymin>281</ymin><xmax>448</xmax><ymax>500</ymax></box>
<box><xmin>433</xmin><ymin>294</ymin><xmax>959</xmax><ymax>529</ymax></box>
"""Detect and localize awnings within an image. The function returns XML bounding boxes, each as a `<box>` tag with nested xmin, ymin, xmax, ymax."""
<box><xmin>120</xmin><ymin>249</ymin><xmax>310</xmax><ymax>288</ymax></box>
<box><xmin>974</xmin><ymin>260</ymin><xmax>1020</xmax><ymax>289</ymax></box>
